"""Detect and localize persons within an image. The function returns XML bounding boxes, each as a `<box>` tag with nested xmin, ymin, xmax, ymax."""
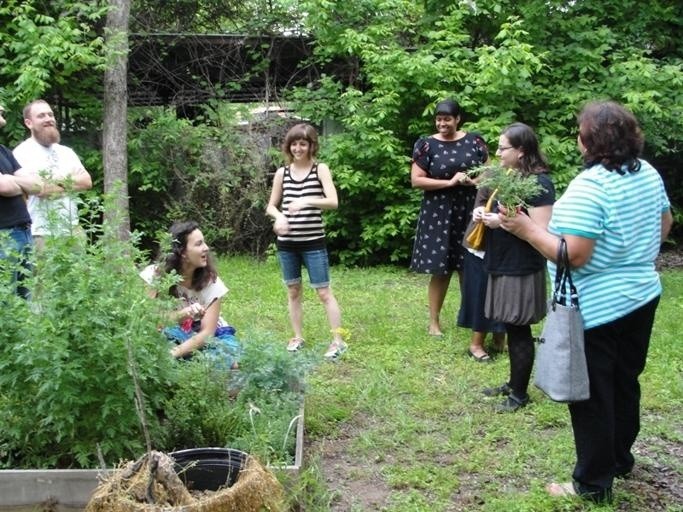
<box><xmin>14</xmin><ymin>99</ymin><xmax>93</xmax><ymax>274</ymax></box>
<box><xmin>409</xmin><ymin>99</ymin><xmax>489</xmax><ymax>337</ymax></box>
<box><xmin>139</xmin><ymin>222</ymin><xmax>243</xmax><ymax>405</ymax></box>
<box><xmin>0</xmin><ymin>105</ymin><xmax>41</xmax><ymax>302</ymax></box>
<box><xmin>496</xmin><ymin>101</ymin><xmax>674</xmax><ymax>507</ymax></box>
<box><xmin>472</xmin><ymin>123</ymin><xmax>555</xmax><ymax>413</ymax></box>
<box><xmin>266</xmin><ymin>123</ymin><xmax>349</xmax><ymax>361</ymax></box>
<box><xmin>456</xmin><ymin>159</ymin><xmax>511</xmax><ymax>363</ymax></box>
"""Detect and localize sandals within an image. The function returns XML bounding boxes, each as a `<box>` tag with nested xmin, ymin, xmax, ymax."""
<box><xmin>467</xmin><ymin>346</ymin><xmax>494</xmax><ymax>365</ymax></box>
<box><xmin>427</xmin><ymin>325</ymin><xmax>446</xmax><ymax>340</ymax></box>
<box><xmin>488</xmin><ymin>343</ymin><xmax>511</xmax><ymax>354</ymax></box>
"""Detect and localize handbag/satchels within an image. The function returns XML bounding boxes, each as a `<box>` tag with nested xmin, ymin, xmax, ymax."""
<box><xmin>531</xmin><ymin>297</ymin><xmax>593</xmax><ymax>403</ymax></box>
<box><xmin>465</xmin><ymin>203</ymin><xmax>492</xmax><ymax>251</ymax></box>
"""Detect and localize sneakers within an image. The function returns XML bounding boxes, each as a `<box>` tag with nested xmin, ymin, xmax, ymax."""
<box><xmin>616</xmin><ymin>463</ymin><xmax>635</xmax><ymax>479</ymax></box>
<box><xmin>493</xmin><ymin>392</ymin><xmax>529</xmax><ymax>415</ymax></box>
<box><xmin>324</xmin><ymin>340</ymin><xmax>348</xmax><ymax>361</ymax></box>
<box><xmin>286</xmin><ymin>338</ymin><xmax>306</xmax><ymax>353</ymax></box>
<box><xmin>483</xmin><ymin>382</ymin><xmax>512</xmax><ymax>397</ymax></box>
<box><xmin>544</xmin><ymin>483</ymin><xmax>612</xmax><ymax>505</ymax></box>
